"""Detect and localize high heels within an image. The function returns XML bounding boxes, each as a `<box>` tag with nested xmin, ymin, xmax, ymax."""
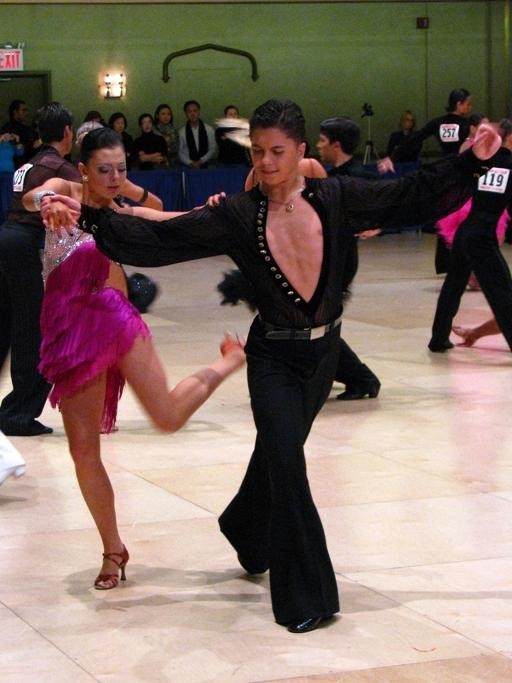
<box><xmin>95</xmin><ymin>545</ymin><xmax>129</xmax><ymax>589</ymax></box>
<box><xmin>452</xmin><ymin>326</ymin><xmax>473</xmax><ymax>347</ymax></box>
<box><xmin>220</xmin><ymin>332</ymin><xmax>240</xmax><ymax>354</ymax></box>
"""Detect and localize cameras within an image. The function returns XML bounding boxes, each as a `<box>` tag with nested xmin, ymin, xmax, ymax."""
<box><xmin>360</xmin><ymin>102</ymin><xmax>374</xmax><ymax>118</ymax></box>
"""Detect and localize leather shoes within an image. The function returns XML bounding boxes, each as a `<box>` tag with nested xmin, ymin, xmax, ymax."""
<box><xmin>338</xmin><ymin>390</ymin><xmax>379</xmax><ymax>400</ymax></box>
<box><xmin>288</xmin><ymin>618</ymin><xmax>320</xmax><ymax>633</ymax></box>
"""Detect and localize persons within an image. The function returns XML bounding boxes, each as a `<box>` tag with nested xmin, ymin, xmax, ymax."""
<box><xmin>40</xmin><ymin>96</ymin><xmax>501</xmax><ymax>636</ymax></box>
<box><xmin>376</xmin><ymin>90</ymin><xmax>510</xmax><ymax>362</ymax></box>
<box><xmin>0</xmin><ymin>89</ymin><xmax>512</xmax><ymax>176</ymax></box>
<box><xmin>0</xmin><ymin>101</ymin><xmax>162</xmax><ymax>437</ymax></box>
<box><xmin>197</xmin><ymin>118</ymin><xmax>393</xmax><ymax>402</ymax></box>
<box><xmin>19</xmin><ymin>126</ymin><xmax>251</xmax><ymax>590</ymax></box>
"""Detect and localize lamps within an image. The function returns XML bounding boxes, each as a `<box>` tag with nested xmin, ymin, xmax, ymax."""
<box><xmin>118</xmin><ymin>74</ymin><xmax>125</xmax><ymax>96</ymax></box>
<box><xmin>104</xmin><ymin>74</ymin><xmax>111</xmax><ymax>97</ymax></box>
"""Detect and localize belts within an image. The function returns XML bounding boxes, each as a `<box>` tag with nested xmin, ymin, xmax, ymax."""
<box><xmin>266</xmin><ymin>315</ymin><xmax>341</xmax><ymax>341</ymax></box>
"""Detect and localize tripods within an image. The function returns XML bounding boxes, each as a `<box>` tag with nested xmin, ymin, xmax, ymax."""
<box><xmin>359</xmin><ymin>119</ymin><xmax>382</xmax><ymax>164</ymax></box>
